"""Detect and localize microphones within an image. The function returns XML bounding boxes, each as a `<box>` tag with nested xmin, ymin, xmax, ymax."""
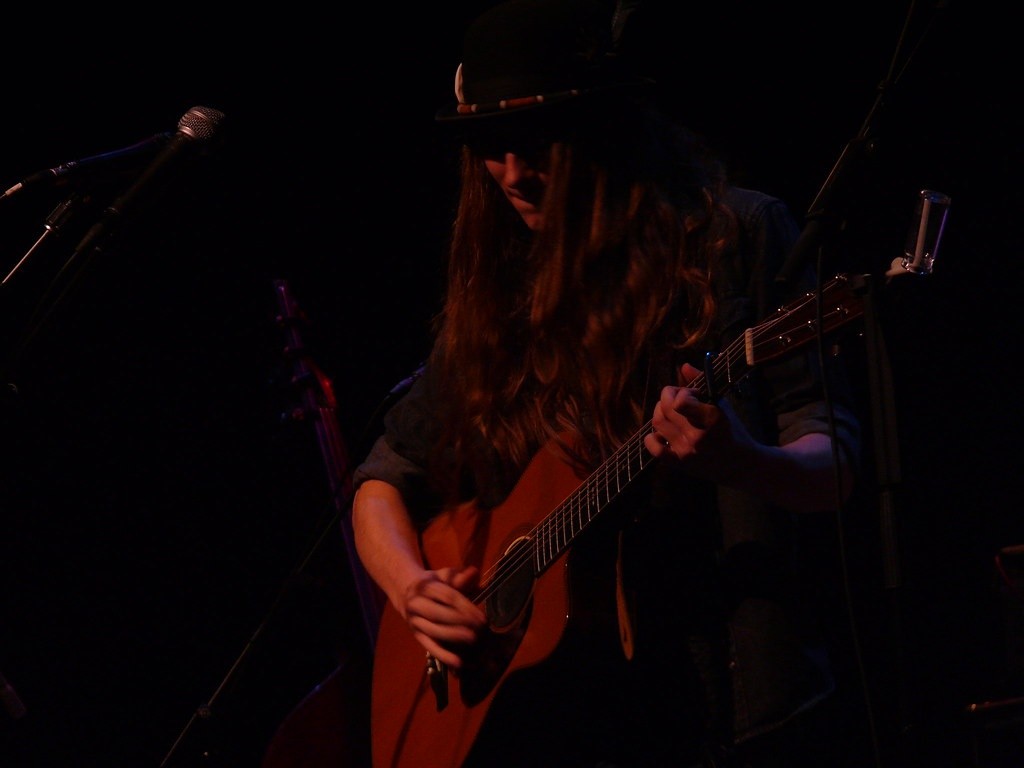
<box><xmin>40</xmin><ymin>106</ymin><xmax>227</xmax><ymax>273</ymax></box>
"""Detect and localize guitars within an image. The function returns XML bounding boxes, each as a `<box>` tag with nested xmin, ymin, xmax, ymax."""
<box><xmin>270</xmin><ymin>278</ymin><xmax>379</xmax><ymax>642</ymax></box>
<box><xmin>370</xmin><ymin>194</ymin><xmax>950</xmax><ymax>768</ymax></box>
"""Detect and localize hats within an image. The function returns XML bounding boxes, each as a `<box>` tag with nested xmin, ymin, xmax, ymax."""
<box><xmin>434</xmin><ymin>3</ymin><xmax>640</xmax><ymax>122</ymax></box>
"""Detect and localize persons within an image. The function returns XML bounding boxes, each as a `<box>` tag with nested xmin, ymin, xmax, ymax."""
<box><xmin>346</xmin><ymin>15</ymin><xmax>868</xmax><ymax>768</ymax></box>
